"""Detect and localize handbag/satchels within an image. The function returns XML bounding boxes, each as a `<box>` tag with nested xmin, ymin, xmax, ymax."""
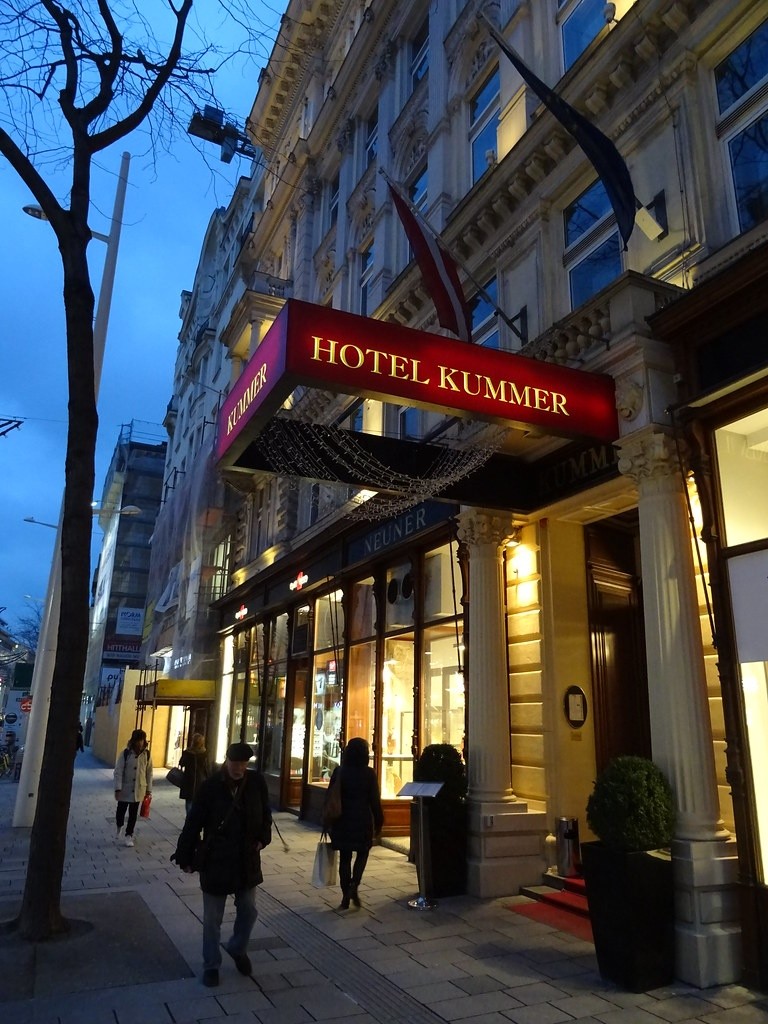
<box><xmin>319</xmin><ymin>767</ymin><xmax>342</xmax><ymax>827</ymax></box>
<box><xmin>140</xmin><ymin>792</ymin><xmax>152</xmax><ymax>818</ymax></box>
<box><xmin>312</xmin><ymin>828</ymin><xmax>337</xmax><ymax>886</ymax></box>
<box><xmin>166</xmin><ymin>765</ymin><xmax>194</xmax><ymax>793</ymax></box>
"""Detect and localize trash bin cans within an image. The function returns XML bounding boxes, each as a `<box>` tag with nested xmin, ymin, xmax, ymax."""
<box><xmin>12</xmin><ymin>747</ymin><xmax>24</xmax><ymax>782</ymax></box>
<box><xmin>556</xmin><ymin>816</ymin><xmax>581</xmax><ymax>878</ymax></box>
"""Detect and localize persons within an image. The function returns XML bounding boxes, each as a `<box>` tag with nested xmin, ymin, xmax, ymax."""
<box><xmin>76</xmin><ymin>722</ymin><xmax>84</xmax><ymax>756</ymax></box>
<box><xmin>321</xmin><ymin>738</ymin><xmax>383</xmax><ymax>908</ymax></box>
<box><xmin>113</xmin><ymin>730</ymin><xmax>152</xmax><ymax>846</ymax></box>
<box><xmin>174</xmin><ymin>743</ymin><xmax>272</xmax><ymax>986</ymax></box>
<box><xmin>179</xmin><ymin>733</ymin><xmax>211</xmax><ymax>818</ymax></box>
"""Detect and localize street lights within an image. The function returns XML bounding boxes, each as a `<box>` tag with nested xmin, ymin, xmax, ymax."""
<box><xmin>9</xmin><ymin>146</ymin><xmax>133</xmax><ymax>827</ymax></box>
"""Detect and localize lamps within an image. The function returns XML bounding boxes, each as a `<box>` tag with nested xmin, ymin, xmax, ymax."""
<box><xmin>23</xmin><ymin>516</ymin><xmax>58</xmax><ymax>530</ymax></box>
<box><xmin>93</xmin><ymin>505</ymin><xmax>142</xmax><ymax>516</ymax></box>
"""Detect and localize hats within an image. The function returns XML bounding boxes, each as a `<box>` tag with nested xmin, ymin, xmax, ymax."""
<box><xmin>226</xmin><ymin>744</ymin><xmax>254</xmax><ymax>762</ymax></box>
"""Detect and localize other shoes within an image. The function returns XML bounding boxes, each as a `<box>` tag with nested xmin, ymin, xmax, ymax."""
<box><xmin>340</xmin><ymin>900</ymin><xmax>350</xmax><ymax>909</ymax></box>
<box><xmin>350</xmin><ymin>888</ymin><xmax>361</xmax><ymax>908</ymax></box>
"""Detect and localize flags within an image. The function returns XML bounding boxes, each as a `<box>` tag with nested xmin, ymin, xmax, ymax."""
<box><xmin>386</xmin><ymin>181</ymin><xmax>472</xmax><ymax>344</ymax></box>
<box><xmin>485</xmin><ymin>29</ymin><xmax>635</xmax><ymax>252</ymax></box>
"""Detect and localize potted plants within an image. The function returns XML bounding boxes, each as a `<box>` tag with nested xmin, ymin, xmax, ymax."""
<box><xmin>410</xmin><ymin>742</ymin><xmax>469</xmax><ymax>898</ymax></box>
<box><xmin>579</xmin><ymin>755</ymin><xmax>678</xmax><ymax>993</ymax></box>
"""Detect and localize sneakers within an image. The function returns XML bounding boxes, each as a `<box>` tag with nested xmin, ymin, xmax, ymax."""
<box><xmin>203</xmin><ymin>969</ymin><xmax>219</xmax><ymax>987</ymax></box>
<box><xmin>228</xmin><ymin>948</ymin><xmax>253</xmax><ymax>975</ymax></box>
<box><xmin>124</xmin><ymin>835</ymin><xmax>134</xmax><ymax>847</ymax></box>
<box><xmin>116</xmin><ymin>825</ymin><xmax>124</xmax><ymax>835</ymax></box>
